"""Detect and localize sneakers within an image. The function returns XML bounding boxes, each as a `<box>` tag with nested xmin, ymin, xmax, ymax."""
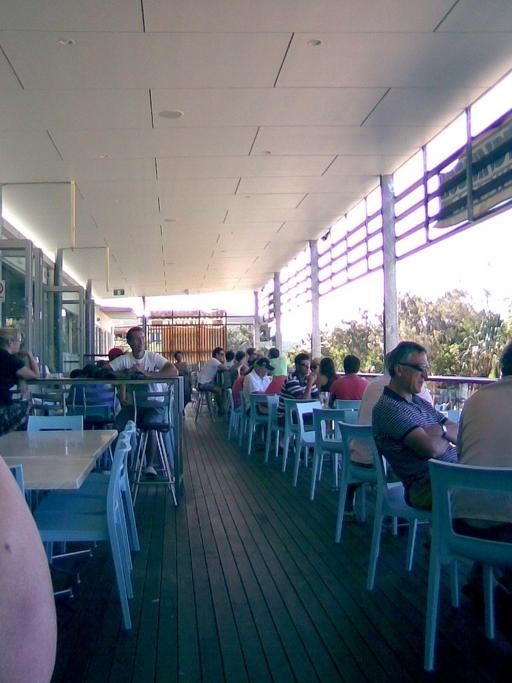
<box><xmin>280</xmin><ymin>438</ymin><xmax>293</xmax><ymax>453</ymax></box>
<box><xmin>144</xmin><ymin>465</ymin><xmax>157</xmax><ymax>480</ymax></box>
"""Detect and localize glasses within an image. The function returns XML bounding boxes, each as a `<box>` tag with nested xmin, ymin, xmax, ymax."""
<box><xmin>398</xmin><ymin>360</ymin><xmax>429</xmax><ymax>372</ymax></box>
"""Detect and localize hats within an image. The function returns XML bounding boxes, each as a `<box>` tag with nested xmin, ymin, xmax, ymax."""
<box><xmin>256</xmin><ymin>357</ymin><xmax>275</xmax><ymax>371</ymax></box>
<box><xmin>108</xmin><ymin>348</ymin><xmax>123</xmax><ymax>356</ymax></box>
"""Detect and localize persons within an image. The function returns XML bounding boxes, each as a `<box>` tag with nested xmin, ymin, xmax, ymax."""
<box><xmin>374</xmin><ymin>341</ymin><xmax>467</xmax><ymax>560</ymax></box>
<box><xmin>329</xmin><ymin>355</ymin><xmax>368</xmax><ymax>407</ymax></box>
<box><xmin>172</xmin><ymin>350</ymin><xmax>191</xmax><ymax>376</ymax></box>
<box><xmin>67</xmin><ymin>348</ymin><xmax>129</xmax><ymax>431</ymax></box>
<box><xmin>277</xmin><ymin>353</ymin><xmax>341</xmax><ymax>462</ymax></box>
<box><xmin>354</xmin><ymin>349</ymin><xmax>434</xmax><ymax>512</ymax></box>
<box><xmin>221</xmin><ymin>348</ymin><xmax>288</xmax><ymax>416</ymax></box>
<box><xmin>2</xmin><ymin>327</ymin><xmax>40</xmax><ymax>431</ymax></box>
<box><xmin>452</xmin><ymin>340</ymin><xmax>511</xmax><ymax>615</ymax></box>
<box><xmin>197</xmin><ymin>346</ymin><xmax>229</xmax><ymax>417</ymax></box>
<box><xmin>102</xmin><ymin>327</ymin><xmax>179</xmax><ymax>477</ymax></box>
<box><xmin>0</xmin><ymin>457</ymin><xmax>58</xmax><ymax>683</ymax></box>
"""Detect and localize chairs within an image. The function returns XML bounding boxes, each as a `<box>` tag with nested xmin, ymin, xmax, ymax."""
<box><xmin>335</xmin><ymin>419</ymin><xmax>463</xmax><ymax>606</ymax></box>
<box><xmin>0</xmin><ymin>362</ymin><xmax>198</xmax><ymax>642</ymax></box>
<box><xmin>284</xmin><ymin>386</ymin><xmax>401</xmax><ymax>536</ymax></box>
<box><xmin>425</xmin><ymin>458</ymin><xmax>512</xmax><ymax>673</ymax></box>
<box><xmin>189</xmin><ymin>355</ymin><xmax>285</xmax><ymax>465</ymax></box>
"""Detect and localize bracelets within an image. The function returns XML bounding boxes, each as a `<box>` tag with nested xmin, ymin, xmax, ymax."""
<box><xmin>441</xmin><ymin>423</ymin><xmax>449</xmax><ymax>439</ymax></box>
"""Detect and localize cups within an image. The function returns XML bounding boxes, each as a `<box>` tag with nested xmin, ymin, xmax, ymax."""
<box><xmin>320</xmin><ymin>391</ymin><xmax>329</xmax><ymax>408</ymax></box>
<box><xmin>40</xmin><ymin>364</ymin><xmax>50</xmax><ymax>378</ymax></box>
